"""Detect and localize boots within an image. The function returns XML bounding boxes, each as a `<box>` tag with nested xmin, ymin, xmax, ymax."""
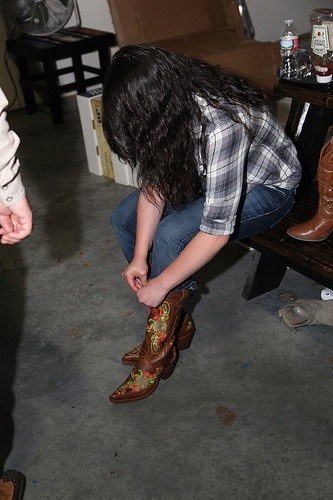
<box><xmin>286</xmin><ymin>137</ymin><xmax>333</xmax><ymax>241</ymax></box>
<box><xmin>278</xmin><ymin>298</ymin><xmax>333</xmax><ymax>328</ymax></box>
<box><xmin>121</xmin><ymin>288</ymin><xmax>198</xmax><ymax>366</ymax></box>
<box><xmin>108</xmin><ymin>289</ymin><xmax>190</xmax><ymax>403</ymax></box>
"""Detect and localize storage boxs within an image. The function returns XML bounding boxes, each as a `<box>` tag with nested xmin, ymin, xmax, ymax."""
<box><xmin>75</xmin><ymin>88</ymin><xmax>141</xmax><ymax>188</ymax></box>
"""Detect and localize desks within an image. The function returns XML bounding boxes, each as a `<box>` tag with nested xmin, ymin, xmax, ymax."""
<box><xmin>273</xmin><ymin>76</ymin><xmax>333</xmax><ymax>199</ymax></box>
<box><xmin>5</xmin><ymin>25</ymin><xmax>117</xmax><ymax>125</ymax></box>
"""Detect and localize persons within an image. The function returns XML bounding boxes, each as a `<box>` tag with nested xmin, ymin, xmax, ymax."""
<box><xmin>102</xmin><ymin>44</ymin><xmax>304</xmax><ymax>403</ymax></box>
<box><xmin>0</xmin><ymin>85</ymin><xmax>34</xmax><ymax>500</ymax></box>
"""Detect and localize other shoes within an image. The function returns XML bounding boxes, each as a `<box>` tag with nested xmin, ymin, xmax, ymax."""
<box><xmin>0</xmin><ymin>470</ymin><xmax>26</xmax><ymax>500</ymax></box>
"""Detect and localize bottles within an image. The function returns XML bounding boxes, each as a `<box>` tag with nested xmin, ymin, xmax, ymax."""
<box><xmin>309</xmin><ymin>7</ymin><xmax>333</xmax><ymax>83</ymax></box>
<box><xmin>278</xmin><ymin>19</ymin><xmax>299</xmax><ymax>80</ymax></box>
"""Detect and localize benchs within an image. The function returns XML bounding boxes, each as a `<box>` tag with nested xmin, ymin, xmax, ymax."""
<box><xmin>234</xmin><ymin>201</ymin><xmax>333</xmax><ymax>302</ymax></box>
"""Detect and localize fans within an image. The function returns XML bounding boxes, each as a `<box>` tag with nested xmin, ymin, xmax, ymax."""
<box><xmin>0</xmin><ymin>0</ymin><xmax>82</xmax><ymax>37</ymax></box>
